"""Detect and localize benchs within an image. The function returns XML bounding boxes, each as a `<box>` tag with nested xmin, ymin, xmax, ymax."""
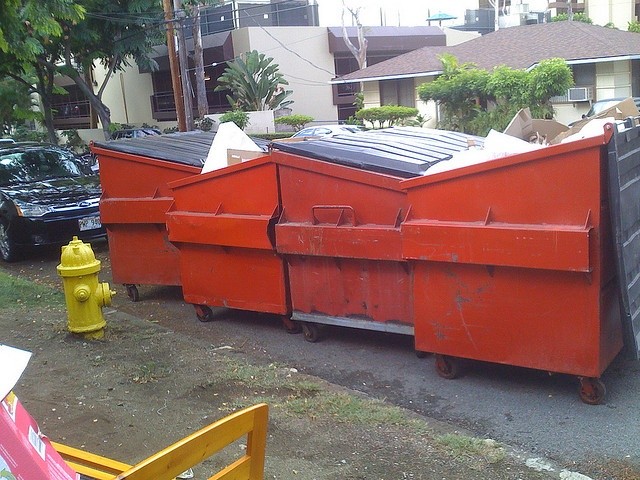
<box><xmin>40</xmin><ymin>403</ymin><xmax>269</xmax><ymax>479</ymax></box>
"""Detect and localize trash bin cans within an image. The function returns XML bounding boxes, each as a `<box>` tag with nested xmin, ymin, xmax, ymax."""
<box><xmin>165</xmin><ymin>137</ymin><xmax>300</xmax><ymax>335</ymax></box>
<box><xmin>399</xmin><ymin>110</ymin><xmax>640</xmax><ymax>405</ymax></box>
<box><xmin>88</xmin><ymin>130</ymin><xmax>272</xmax><ymax>301</ymax></box>
<box><xmin>271</xmin><ymin>125</ymin><xmax>487</xmax><ymax>359</ymax></box>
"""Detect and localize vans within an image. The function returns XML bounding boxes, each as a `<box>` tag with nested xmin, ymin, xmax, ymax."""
<box><xmin>109</xmin><ymin>128</ymin><xmax>162</xmax><ymax>140</ymax></box>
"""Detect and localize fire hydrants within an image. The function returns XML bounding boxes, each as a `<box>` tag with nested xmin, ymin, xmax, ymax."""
<box><xmin>55</xmin><ymin>234</ymin><xmax>117</xmax><ymax>343</ymax></box>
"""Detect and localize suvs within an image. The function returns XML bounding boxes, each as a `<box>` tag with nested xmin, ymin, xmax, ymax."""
<box><xmin>0</xmin><ymin>138</ymin><xmax>107</xmax><ymax>261</ymax></box>
<box><xmin>581</xmin><ymin>97</ymin><xmax>640</xmax><ymax>128</ymax></box>
<box><xmin>290</xmin><ymin>120</ymin><xmax>363</xmax><ymax>138</ymax></box>
